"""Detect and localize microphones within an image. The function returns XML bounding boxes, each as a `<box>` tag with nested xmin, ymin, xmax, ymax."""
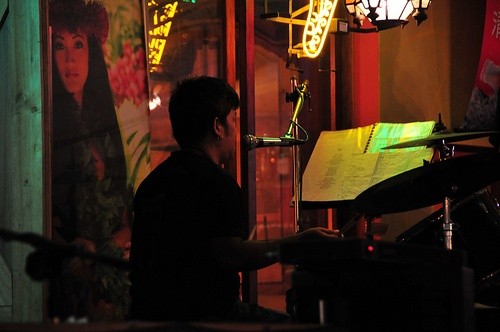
<box><xmin>242</xmin><ymin>134</ymin><xmax>304</xmax><ymax>149</ymax></box>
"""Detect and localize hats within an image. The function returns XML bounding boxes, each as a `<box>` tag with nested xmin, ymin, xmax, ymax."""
<box><xmin>47</xmin><ymin>0</ymin><xmax>110</xmax><ymax>44</ymax></box>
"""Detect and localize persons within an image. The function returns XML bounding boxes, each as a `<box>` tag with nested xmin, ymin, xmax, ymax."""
<box><xmin>130</xmin><ymin>76</ymin><xmax>346</xmax><ymax>325</ymax></box>
<box><xmin>50</xmin><ymin>0</ymin><xmax>133</xmax><ymax>259</ymax></box>
